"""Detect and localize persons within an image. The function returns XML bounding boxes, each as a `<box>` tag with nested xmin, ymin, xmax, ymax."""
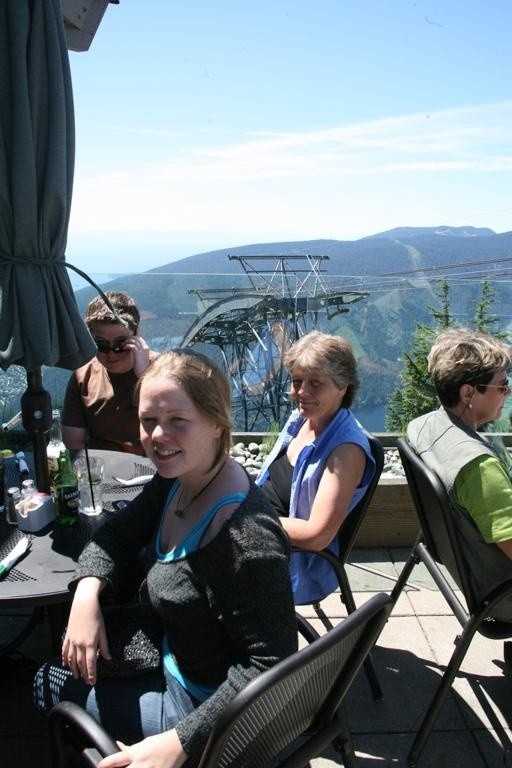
<box><xmin>255</xmin><ymin>329</ymin><xmax>377</xmax><ymax>607</ymax></box>
<box><xmin>60</xmin><ymin>289</ymin><xmax>163</xmax><ymax>457</ymax></box>
<box><xmin>31</xmin><ymin>349</ymin><xmax>298</xmax><ymax>768</ymax></box>
<box><xmin>405</xmin><ymin>326</ymin><xmax>512</xmax><ymax>624</ymax></box>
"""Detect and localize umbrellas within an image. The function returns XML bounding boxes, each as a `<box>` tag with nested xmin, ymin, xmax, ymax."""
<box><xmin>0</xmin><ymin>2</ymin><xmax>98</xmax><ymax>492</ymax></box>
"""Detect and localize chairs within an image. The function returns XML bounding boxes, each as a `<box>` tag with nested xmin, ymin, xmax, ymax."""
<box><xmin>45</xmin><ymin>589</ymin><xmax>394</xmax><ymax>766</ymax></box>
<box><xmin>271</xmin><ymin>426</ymin><xmax>387</xmax><ymax>703</ymax></box>
<box><xmin>377</xmin><ymin>433</ymin><xmax>512</xmax><ymax>766</ymax></box>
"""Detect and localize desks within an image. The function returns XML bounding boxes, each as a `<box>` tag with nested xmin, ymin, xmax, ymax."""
<box><xmin>0</xmin><ymin>441</ymin><xmax>165</xmax><ymax>705</ymax></box>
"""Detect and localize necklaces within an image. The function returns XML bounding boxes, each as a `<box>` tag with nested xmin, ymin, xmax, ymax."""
<box><xmin>174</xmin><ymin>459</ymin><xmax>228</xmax><ymax>518</ymax></box>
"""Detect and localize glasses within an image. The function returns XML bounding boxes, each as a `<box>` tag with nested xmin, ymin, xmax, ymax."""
<box><xmin>479</xmin><ymin>378</ymin><xmax>509</xmax><ymax>391</ymax></box>
<box><xmin>96</xmin><ymin>343</ymin><xmax>128</xmax><ymax>353</ymax></box>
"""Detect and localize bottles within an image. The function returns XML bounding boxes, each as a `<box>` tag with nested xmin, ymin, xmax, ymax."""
<box><xmin>52</xmin><ymin>450</ymin><xmax>79</xmax><ymax>526</ymax></box>
<box><xmin>45</xmin><ymin>409</ymin><xmax>68</xmax><ymax>492</ymax></box>
<box><xmin>0</xmin><ymin>448</ymin><xmax>15</xmax><ymax>510</ymax></box>
<box><xmin>6</xmin><ymin>486</ymin><xmax>23</xmax><ymax>526</ymax></box>
<box><xmin>21</xmin><ymin>480</ymin><xmax>40</xmax><ymax>499</ymax></box>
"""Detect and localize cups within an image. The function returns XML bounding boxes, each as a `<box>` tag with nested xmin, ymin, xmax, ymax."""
<box><xmin>73</xmin><ymin>456</ymin><xmax>106</xmax><ymax>517</ymax></box>
